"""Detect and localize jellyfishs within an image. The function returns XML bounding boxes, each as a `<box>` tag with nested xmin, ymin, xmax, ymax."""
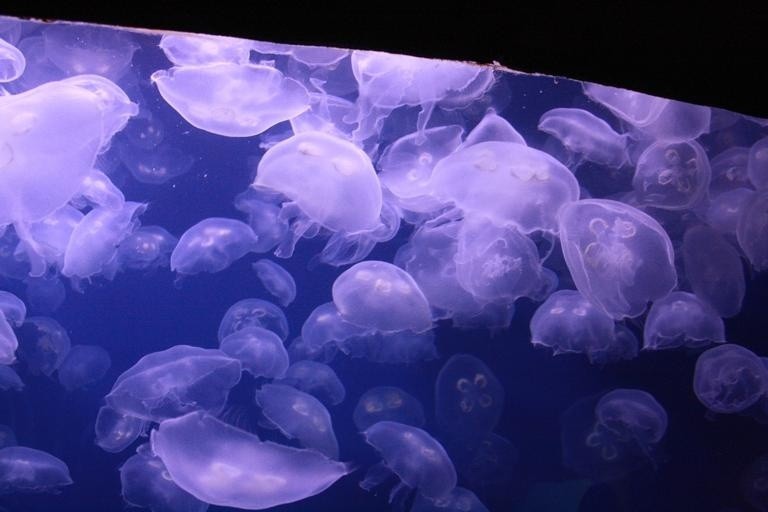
<box><xmin>0</xmin><ymin>17</ymin><xmax>768</xmax><ymax>512</ymax></box>
